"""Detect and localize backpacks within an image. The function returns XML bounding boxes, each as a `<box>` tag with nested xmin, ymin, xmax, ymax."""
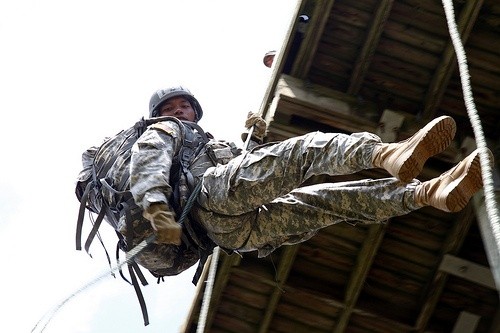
<box><xmin>75</xmin><ymin>116</ymin><xmax>213</xmax><ymax>278</ymax></box>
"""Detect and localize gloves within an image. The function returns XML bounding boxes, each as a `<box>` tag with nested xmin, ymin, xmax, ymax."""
<box><xmin>148</xmin><ymin>204</ymin><xmax>182</xmax><ymax>246</ymax></box>
<box><xmin>245</xmin><ymin>111</ymin><xmax>267</xmax><ymax>138</ymax></box>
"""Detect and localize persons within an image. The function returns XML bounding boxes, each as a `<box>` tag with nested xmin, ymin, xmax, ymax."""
<box><xmin>129</xmin><ymin>87</ymin><xmax>496</xmax><ymax>258</ymax></box>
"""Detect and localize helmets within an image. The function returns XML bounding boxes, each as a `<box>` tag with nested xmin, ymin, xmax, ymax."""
<box><xmin>148</xmin><ymin>86</ymin><xmax>203</xmax><ymax>123</ymax></box>
<box><xmin>263</xmin><ymin>50</ymin><xmax>276</xmax><ymax>68</ymax></box>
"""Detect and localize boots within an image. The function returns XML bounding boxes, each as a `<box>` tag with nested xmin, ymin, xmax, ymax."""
<box><xmin>372</xmin><ymin>115</ymin><xmax>456</xmax><ymax>184</ymax></box>
<box><xmin>414</xmin><ymin>147</ymin><xmax>494</xmax><ymax>212</ymax></box>
